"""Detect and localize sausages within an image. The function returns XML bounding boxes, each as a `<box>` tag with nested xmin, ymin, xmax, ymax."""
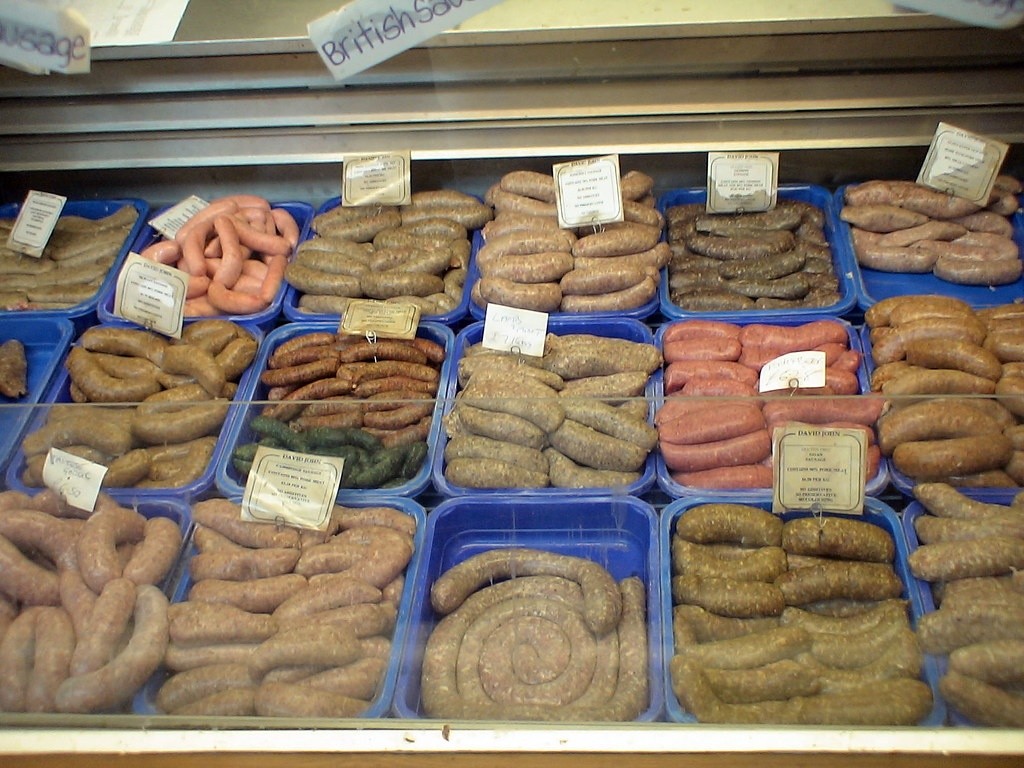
<box><xmin>3</xmin><ymin>167</ymin><xmax>1024</xmax><ymax>728</ymax></box>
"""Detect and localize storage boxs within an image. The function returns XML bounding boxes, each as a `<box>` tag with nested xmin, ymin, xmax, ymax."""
<box><xmin>282</xmin><ymin>191</ymin><xmax>485</xmax><ymax>323</ymax></box>
<box><xmin>832</xmin><ymin>182</ymin><xmax>1024</xmax><ymax>315</ymax></box>
<box><xmin>389</xmin><ymin>496</ymin><xmax>666</xmax><ymax>720</ymax></box>
<box><xmin>659</xmin><ymin>183</ymin><xmax>859</xmax><ymax>321</ymax></box>
<box><xmin>1</xmin><ymin>496</ymin><xmax>198</xmax><ymax>713</ymax></box>
<box><xmin>130</xmin><ymin>495</ymin><xmax>428</xmax><ymax>718</ymax></box>
<box><xmin>659</xmin><ymin>494</ymin><xmax>948</xmax><ymax>727</ymax></box>
<box><xmin>0</xmin><ymin>196</ymin><xmax>151</xmax><ymax>340</ymax></box>
<box><xmin>469</xmin><ymin>191</ymin><xmax>662</xmax><ymax>323</ymax></box>
<box><xmin>431</xmin><ymin>315</ymin><xmax>658</xmax><ymax>499</ymax></box>
<box><xmin>900</xmin><ymin>495</ymin><xmax>1024</xmax><ymax>730</ymax></box>
<box><xmin>655</xmin><ymin>314</ymin><xmax>891</xmax><ymax>503</ymax></box>
<box><xmin>214</xmin><ymin>318</ymin><xmax>454</xmax><ymax>502</ymax></box>
<box><xmin>5</xmin><ymin>323</ymin><xmax>264</xmax><ymax>495</ymax></box>
<box><xmin>94</xmin><ymin>198</ymin><xmax>315</xmax><ymax>332</ymax></box>
<box><xmin>0</xmin><ymin>316</ymin><xmax>75</xmax><ymax>474</ymax></box>
<box><xmin>859</xmin><ymin>317</ymin><xmax>1023</xmax><ymax>505</ymax></box>
